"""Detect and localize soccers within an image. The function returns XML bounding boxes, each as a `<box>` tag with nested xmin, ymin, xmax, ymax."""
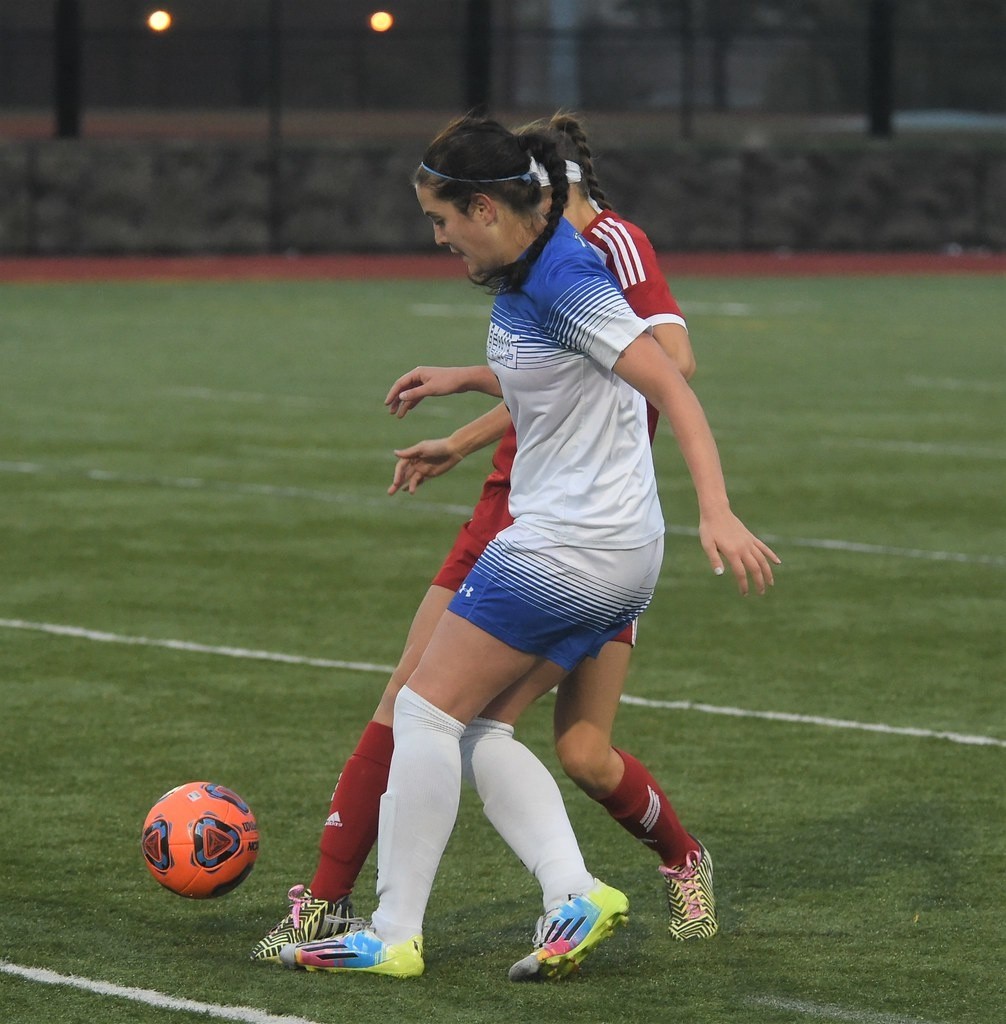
<box><xmin>141</xmin><ymin>782</ymin><xmax>260</xmax><ymax>899</ymax></box>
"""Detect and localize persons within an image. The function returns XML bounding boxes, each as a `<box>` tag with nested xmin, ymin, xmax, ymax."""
<box><xmin>276</xmin><ymin>114</ymin><xmax>787</xmax><ymax>988</ymax></box>
<box><xmin>243</xmin><ymin>104</ymin><xmax>719</xmax><ymax>968</ymax></box>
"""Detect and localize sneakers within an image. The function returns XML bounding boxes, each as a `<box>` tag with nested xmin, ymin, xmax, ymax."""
<box><xmin>278</xmin><ymin>920</ymin><xmax>425</xmax><ymax>980</ymax></box>
<box><xmin>663</xmin><ymin>831</ymin><xmax>718</xmax><ymax>943</ymax></box>
<box><xmin>508</xmin><ymin>877</ymin><xmax>630</xmax><ymax>985</ymax></box>
<box><xmin>249</xmin><ymin>888</ymin><xmax>355</xmax><ymax>962</ymax></box>
